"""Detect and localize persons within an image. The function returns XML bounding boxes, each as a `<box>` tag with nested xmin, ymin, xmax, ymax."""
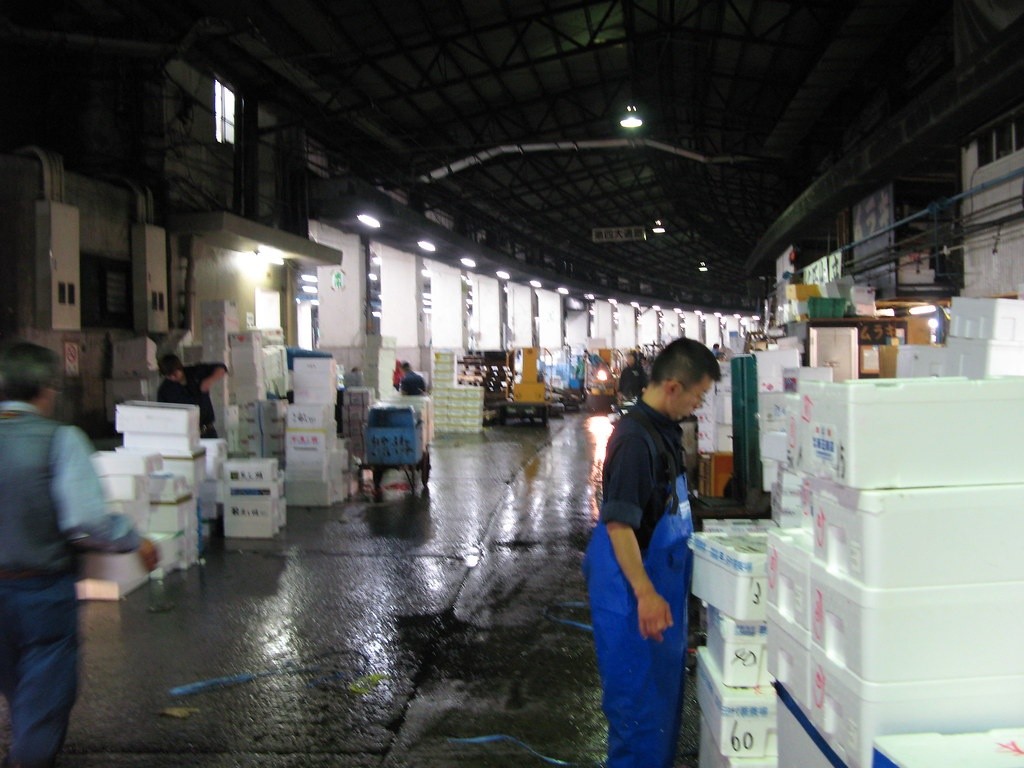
<box><xmin>583</xmin><ymin>338</ymin><xmax>722</xmax><ymax>768</ymax></box>
<box><xmin>158</xmin><ymin>353</ymin><xmax>228</xmax><ymax>437</ymax></box>
<box><xmin>617</xmin><ymin>346</ymin><xmax>647</xmax><ymax>403</ymax></box>
<box><xmin>712</xmin><ymin>343</ymin><xmax>724</xmax><ymax>359</ymax></box>
<box><xmin>393</xmin><ymin>360</ymin><xmax>426</xmax><ymax>395</ymax></box>
<box><xmin>0</xmin><ymin>344</ymin><xmax>160</xmax><ymax>768</ymax></box>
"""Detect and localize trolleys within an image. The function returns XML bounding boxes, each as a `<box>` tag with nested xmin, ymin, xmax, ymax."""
<box><xmin>354</xmin><ymin>448</ymin><xmax>434</xmax><ymax>490</ymax></box>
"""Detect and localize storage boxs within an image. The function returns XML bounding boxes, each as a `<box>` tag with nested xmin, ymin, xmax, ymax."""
<box><xmin>677</xmin><ymin>294</ymin><xmax>1023</xmax><ymax>768</ymax></box>
<box><xmin>70</xmin><ymin>298</ymin><xmax>483</xmax><ymax>600</ymax></box>
